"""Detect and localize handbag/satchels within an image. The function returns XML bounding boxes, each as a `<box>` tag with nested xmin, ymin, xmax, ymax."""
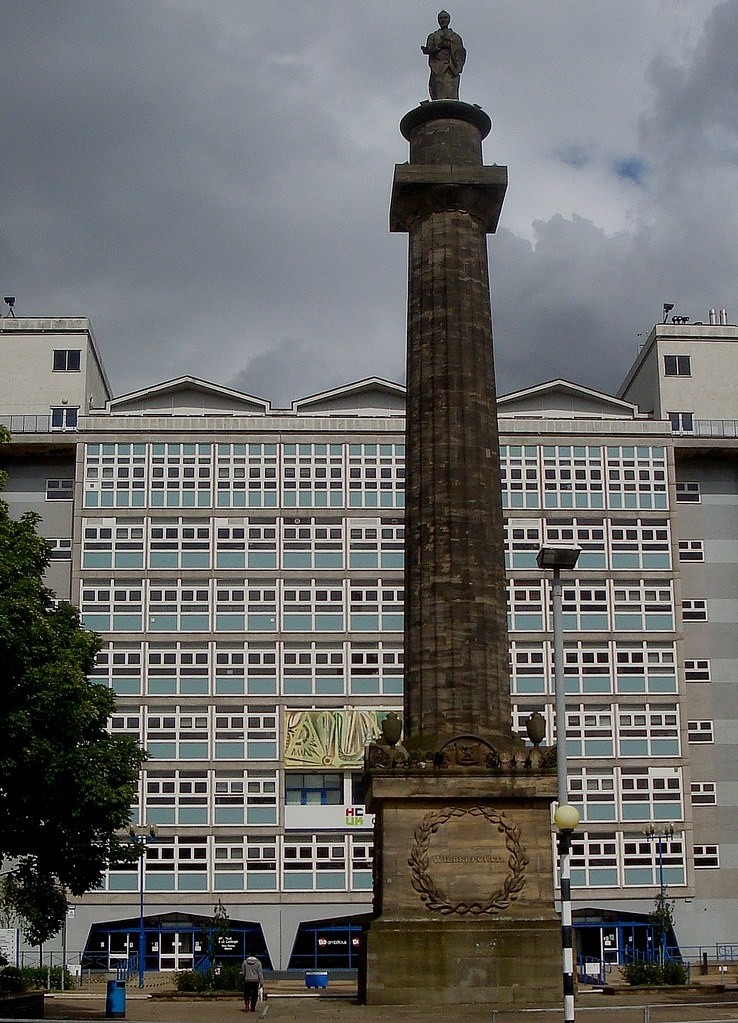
<box><xmin>258</xmin><ymin>986</ymin><xmax>268</xmax><ymax>1003</ymax></box>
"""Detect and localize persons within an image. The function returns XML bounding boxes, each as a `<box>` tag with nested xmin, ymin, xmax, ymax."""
<box><xmin>242</xmin><ymin>949</ymin><xmax>264</xmax><ymax>1012</ymax></box>
<box><xmin>427</xmin><ymin>11</ymin><xmax>466</xmax><ymax>101</ymax></box>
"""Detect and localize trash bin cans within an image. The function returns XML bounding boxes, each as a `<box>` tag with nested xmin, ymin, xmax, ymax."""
<box><xmin>106</xmin><ymin>980</ymin><xmax>126</xmax><ymax>1017</ymax></box>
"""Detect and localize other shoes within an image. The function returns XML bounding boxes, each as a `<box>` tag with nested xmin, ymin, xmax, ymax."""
<box><xmin>245</xmin><ymin>1001</ymin><xmax>250</xmax><ymax>1012</ymax></box>
<box><xmin>250</xmin><ymin>1009</ymin><xmax>255</xmax><ymax>1012</ymax></box>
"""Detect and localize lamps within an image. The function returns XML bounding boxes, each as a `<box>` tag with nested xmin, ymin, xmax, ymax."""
<box><xmin>3</xmin><ymin>296</ymin><xmax>17</xmax><ymax>319</ymax></box>
<box><xmin>662</xmin><ymin>303</ymin><xmax>689</xmax><ymax>324</ymax></box>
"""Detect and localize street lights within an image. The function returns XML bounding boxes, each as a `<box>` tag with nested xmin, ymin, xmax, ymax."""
<box><xmin>128</xmin><ymin>820</ymin><xmax>156</xmax><ymax>987</ymax></box>
<box><xmin>533</xmin><ymin>540</ymin><xmax>585</xmax><ymax>805</ymax></box>
<box><xmin>642</xmin><ymin>822</ymin><xmax>675</xmax><ymax>963</ymax></box>
<box><xmin>554</xmin><ymin>806</ymin><xmax>581</xmax><ymax>1023</ymax></box>
